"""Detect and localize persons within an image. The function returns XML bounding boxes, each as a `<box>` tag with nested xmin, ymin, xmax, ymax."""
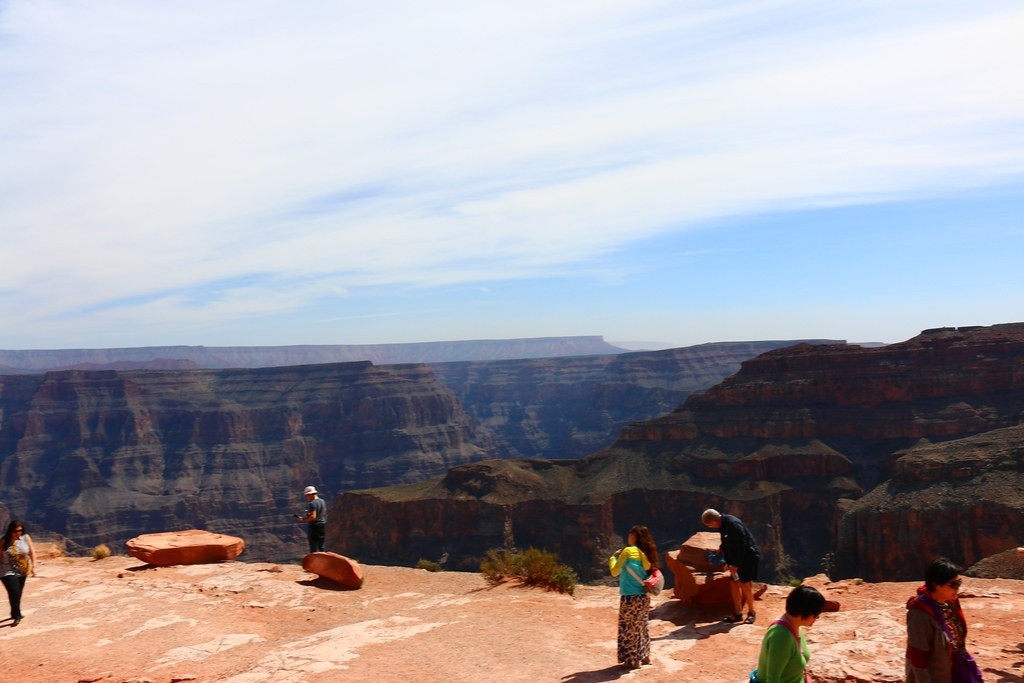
<box><xmin>296</xmin><ymin>486</ymin><xmax>327</xmax><ymax>553</ymax></box>
<box><xmin>905</xmin><ymin>557</ymin><xmax>986</xmax><ymax>683</ymax></box>
<box><xmin>0</xmin><ymin>521</ymin><xmax>36</xmax><ymax>627</ymax></box>
<box><xmin>750</xmin><ymin>585</ymin><xmax>840</xmax><ymax>683</ymax></box>
<box><xmin>702</xmin><ymin>509</ymin><xmax>760</xmax><ymax>624</ymax></box>
<box><xmin>609</xmin><ymin>526</ymin><xmax>657</xmax><ymax>670</ymax></box>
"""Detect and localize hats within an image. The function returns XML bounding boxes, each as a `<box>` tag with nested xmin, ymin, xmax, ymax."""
<box><xmin>303</xmin><ymin>486</ymin><xmax>318</xmax><ymax>496</ymax></box>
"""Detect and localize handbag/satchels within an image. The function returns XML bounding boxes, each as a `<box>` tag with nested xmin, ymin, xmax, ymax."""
<box><xmin>644</xmin><ymin>566</ymin><xmax>665</xmax><ymax>595</ymax></box>
<box><xmin>9</xmin><ymin>554</ymin><xmax>30</xmax><ymax>573</ymax></box>
<box><xmin>952</xmin><ymin>647</ymin><xmax>984</xmax><ymax>683</ymax></box>
<box><xmin>749</xmin><ymin>669</ymin><xmax>758</xmax><ymax>683</ymax></box>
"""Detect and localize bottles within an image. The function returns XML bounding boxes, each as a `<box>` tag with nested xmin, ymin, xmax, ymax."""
<box><xmin>729</xmin><ymin>565</ymin><xmax>739</xmax><ymax>580</ymax></box>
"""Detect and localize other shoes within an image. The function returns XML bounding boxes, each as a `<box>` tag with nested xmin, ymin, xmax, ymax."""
<box><xmin>624</xmin><ymin>658</ymin><xmax>641</xmax><ymax>669</ymax></box>
<box><xmin>744</xmin><ymin>611</ymin><xmax>756</xmax><ymax>624</ymax></box>
<box><xmin>723</xmin><ymin>613</ymin><xmax>743</xmax><ymax>622</ymax></box>
<box><xmin>14</xmin><ymin>618</ymin><xmax>20</xmax><ymax>625</ymax></box>
<box><xmin>641</xmin><ymin>656</ymin><xmax>652</xmax><ymax>664</ymax></box>
<box><xmin>11</xmin><ymin>614</ymin><xmax>23</xmax><ymax>619</ymax></box>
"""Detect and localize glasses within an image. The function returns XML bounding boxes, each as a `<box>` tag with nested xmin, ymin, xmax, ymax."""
<box><xmin>13</xmin><ymin>529</ymin><xmax>22</xmax><ymax>532</ymax></box>
<box><xmin>944</xmin><ymin>578</ymin><xmax>962</xmax><ymax>590</ymax></box>
